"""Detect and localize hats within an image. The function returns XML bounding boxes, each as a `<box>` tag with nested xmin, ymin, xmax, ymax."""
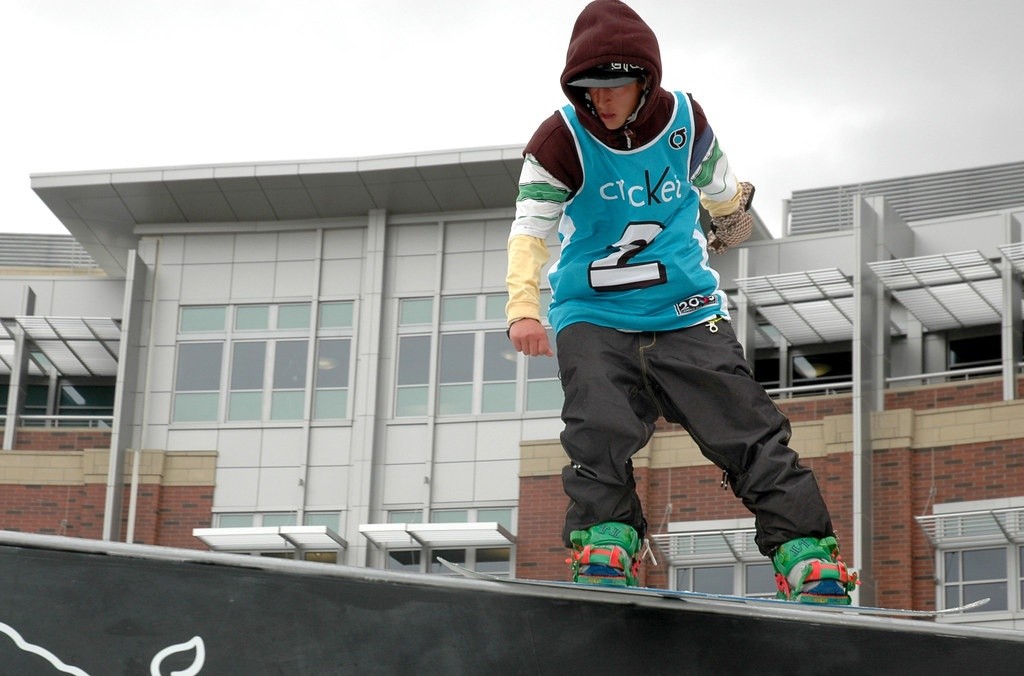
<box><xmin>564</xmin><ymin>62</ymin><xmax>645</xmax><ymax>87</ymax></box>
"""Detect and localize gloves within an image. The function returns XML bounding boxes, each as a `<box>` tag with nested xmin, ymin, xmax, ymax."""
<box><xmin>709</xmin><ymin>209</ymin><xmax>752</xmax><ymax>254</ymax></box>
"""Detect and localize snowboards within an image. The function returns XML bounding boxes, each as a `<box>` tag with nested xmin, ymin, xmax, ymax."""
<box><xmin>435</xmin><ymin>554</ymin><xmax>992</xmax><ymax>616</ymax></box>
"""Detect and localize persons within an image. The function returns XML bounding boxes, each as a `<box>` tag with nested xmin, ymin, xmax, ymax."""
<box><xmin>506</xmin><ymin>0</ymin><xmax>856</xmax><ymax>604</ymax></box>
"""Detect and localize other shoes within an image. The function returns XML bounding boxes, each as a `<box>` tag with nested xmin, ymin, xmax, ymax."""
<box><xmin>569</xmin><ymin>523</ymin><xmax>641</xmax><ymax>587</ymax></box>
<box><xmin>771</xmin><ymin>536</ymin><xmax>858</xmax><ymax>604</ymax></box>
